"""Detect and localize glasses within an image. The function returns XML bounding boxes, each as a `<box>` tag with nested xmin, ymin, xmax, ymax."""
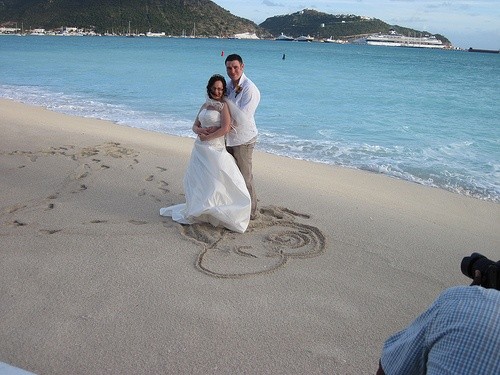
<box><xmin>210</xmin><ymin>87</ymin><xmax>224</xmax><ymax>91</ymax></box>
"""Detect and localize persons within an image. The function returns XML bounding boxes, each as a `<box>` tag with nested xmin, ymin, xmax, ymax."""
<box><xmin>196</xmin><ymin>53</ymin><xmax>260</xmax><ymax>220</ymax></box>
<box><xmin>375</xmin><ymin>258</ymin><xmax>500</xmax><ymax>375</ymax></box>
<box><xmin>191</xmin><ymin>73</ymin><xmax>231</xmax><ymax>222</ymax></box>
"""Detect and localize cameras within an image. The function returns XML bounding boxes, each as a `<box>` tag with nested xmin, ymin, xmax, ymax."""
<box><xmin>461</xmin><ymin>252</ymin><xmax>500</xmax><ymax>291</ymax></box>
